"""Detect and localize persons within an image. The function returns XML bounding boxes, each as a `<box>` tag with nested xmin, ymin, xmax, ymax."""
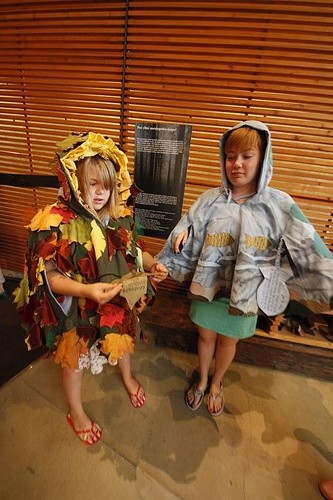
<box><xmin>14</xmin><ymin>131</ymin><xmax>169</xmax><ymax>447</ymax></box>
<box><xmin>319</xmin><ymin>473</ymin><xmax>333</xmax><ymax>499</ymax></box>
<box><xmin>153</xmin><ymin>118</ymin><xmax>333</xmax><ymax>417</ymax></box>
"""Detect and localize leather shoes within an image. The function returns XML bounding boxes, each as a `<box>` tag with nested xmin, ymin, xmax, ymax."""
<box><xmin>319</xmin><ymin>479</ymin><xmax>333</xmax><ymax>500</ymax></box>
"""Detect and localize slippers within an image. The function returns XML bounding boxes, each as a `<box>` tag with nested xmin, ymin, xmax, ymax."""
<box><xmin>66</xmin><ymin>411</ymin><xmax>102</xmax><ymax>446</ymax></box>
<box><xmin>185</xmin><ymin>378</ymin><xmax>225</xmax><ymax>416</ymax></box>
<box><xmin>125</xmin><ymin>377</ymin><xmax>146</xmax><ymax>408</ymax></box>
